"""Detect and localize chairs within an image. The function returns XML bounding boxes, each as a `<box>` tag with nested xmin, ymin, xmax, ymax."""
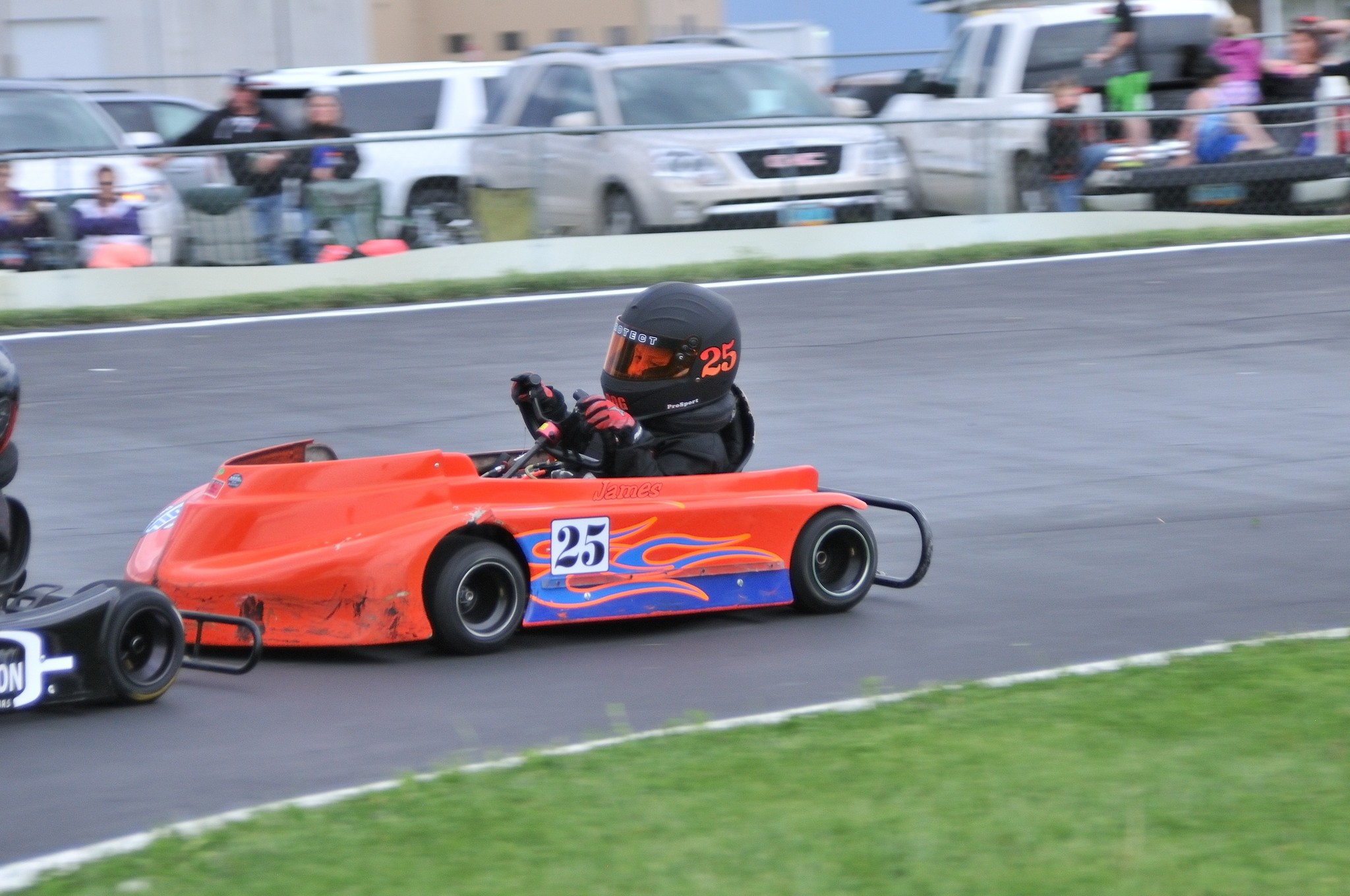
<box><xmin>53</xmin><ymin>194</ymin><xmax>128</xmax><ymax>270</ymax></box>
<box><xmin>174</xmin><ymin>184</ymin><xmax>280</xmax><ymax>268</ymax></box>
<box><xmin>301</xmin><ymin>176</ymin><xmax>436</xmax><ymax>265</ymax></box>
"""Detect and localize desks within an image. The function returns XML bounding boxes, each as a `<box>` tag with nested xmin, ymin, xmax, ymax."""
<box><xmin>1128</xmin><ymin>153</ymin><xmax>1350</xmax><ymax>215</ymax></box>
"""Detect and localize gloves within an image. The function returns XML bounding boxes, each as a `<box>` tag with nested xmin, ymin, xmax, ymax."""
<box><xmin>510</xmin><ymin>371</ymin><xmax>566</xmax><ymax>418</ymax></box>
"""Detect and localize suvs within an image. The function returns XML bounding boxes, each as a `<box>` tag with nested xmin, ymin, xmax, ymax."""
<box><xmin>475</xmin><ymin>34</ymin><xmax>915</xmax><ymax>237</ymax></box>
<box><xmin>881</xmin><ymin>0</ymin><xmax>1247</xmax><ymax>214</ymax></box>
<box><xmin>1</xmin><ymin>72</ymin><xmax>194</xmax><ymax>270</ymax></box>
<box><xmin>240</xmin><ymin>59</ymin><xmax>522</xmax><ymax>248</ymax></box>
<box><xmin>83</xmin><ymin>85</ymin><xmax>246</xmax><ymax>188</ymax></box>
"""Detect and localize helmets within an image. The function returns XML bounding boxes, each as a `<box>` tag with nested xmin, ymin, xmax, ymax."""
<box><xmin>599</xmin><ymin>283</ymin><xmax>741</xmax><ymax>421</ymax></box>
<box><xmin>0</xmin><ymin>342</ymin><xmax>19</xmax><ymax>456</ymax></box>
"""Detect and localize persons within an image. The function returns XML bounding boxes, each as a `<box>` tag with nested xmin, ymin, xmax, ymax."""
<box><xmin>142</xmin><ymin>71</ymin><xmax>285</xmax><ymax>193</ymax></box>
<box><xmin>477</xmin><ymin>280</ymin><xmax>756</xmax><ymax>478</ymax></box>
<box><xmin>1082</xmin><ymin>1</ymin><xmax>1157</xmax><ymax>150</ymax></box>
<box><xmin>1238</xmin><ymin>16</ymin><xmax>1350</xmax><ymax>170</ymax></box>
<box><xmin>1032</xmin><ymin>73</ymin><xmax>1110</xmax><ymax>206</ymax></box>
<box><xmin>242</xmin><ymin>83</ymin><xmax>362</xmax><ymax>197</ymax></box>
<box><xmin>59</xmin><ymin>169</ymin><xmax>140</xmax><ymax>237</ymax></box>
<box><xmin>1164</xmin><ymin>15</ymin><xmax>1279</xmax><ymax>165</ymax></box>
<box><xmin>1</xmin><ymin>162</ymin><xmax>47</xmax><ymax>237</ymax></box>
